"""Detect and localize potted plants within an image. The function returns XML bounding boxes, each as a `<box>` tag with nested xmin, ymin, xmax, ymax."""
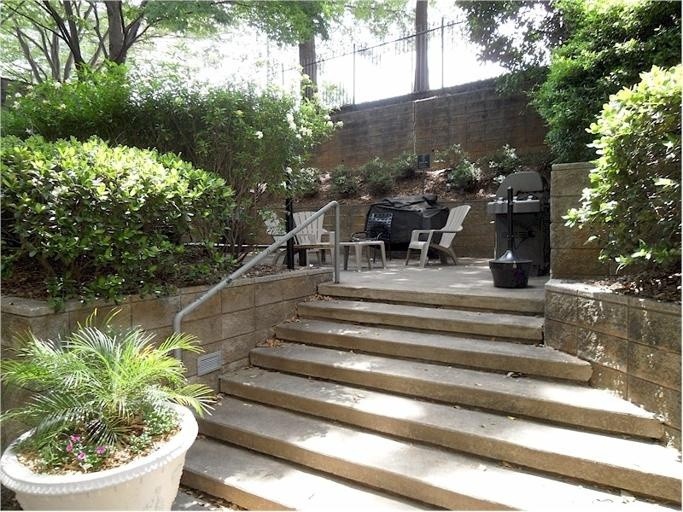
<box><xmin>0</xmin><ymin>308</ymin><xmax>223</xmax><ymax>511</ymax></box>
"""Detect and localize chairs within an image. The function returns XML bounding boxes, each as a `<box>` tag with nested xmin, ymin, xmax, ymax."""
<box><xmin>258</xmin><ymin>205</ymin><xmax>472</xmax><ymax>272</ymax></box>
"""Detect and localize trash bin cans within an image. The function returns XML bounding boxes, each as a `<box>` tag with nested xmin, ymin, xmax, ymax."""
<box><xmin>487</xmin><ymin>171</ymin><xmax>550</xmax><ymax>277</ymax></box>
